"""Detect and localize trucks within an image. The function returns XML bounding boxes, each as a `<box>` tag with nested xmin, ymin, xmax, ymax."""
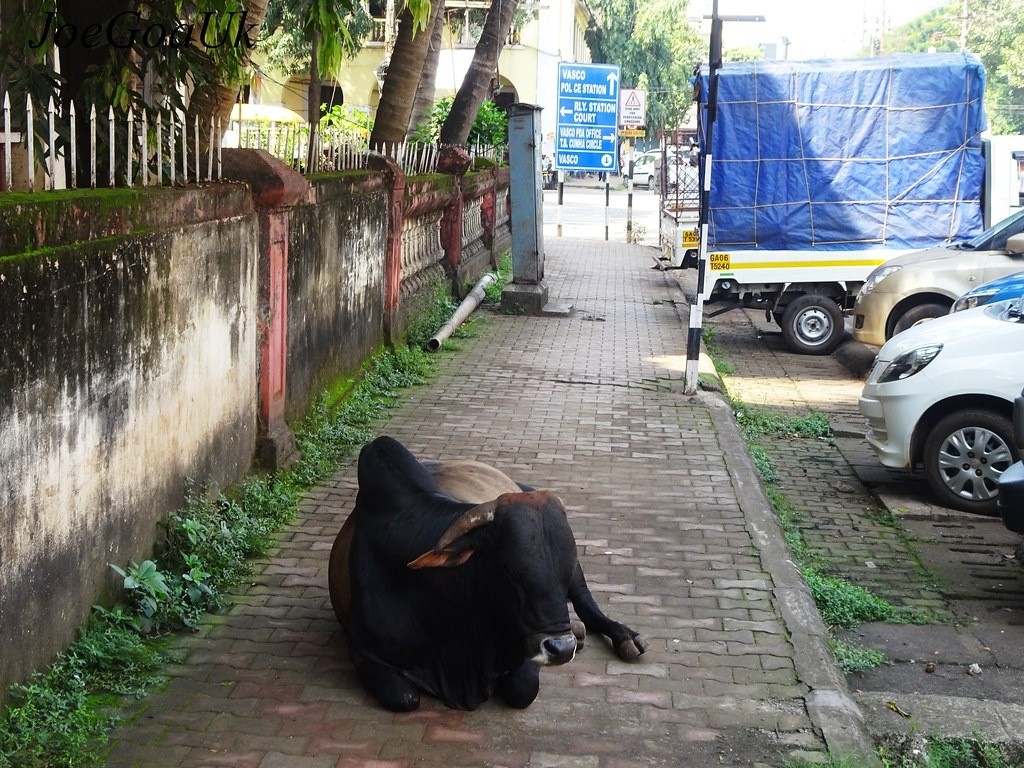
<box><xmin>659</xmin><ymin>135</ymin><xmax>1024</xmax><ymax>356</ymax></box>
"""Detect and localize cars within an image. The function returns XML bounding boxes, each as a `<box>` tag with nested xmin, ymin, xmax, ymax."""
<box><xmin>623</xmin><ymin>146</ymin><xmax>700</xmax><ymax>190</ymax></box>
<box><xmin>832</xmin><ymin>207</ymin><xmax>1024</xmax><ymax>535</ymax></box>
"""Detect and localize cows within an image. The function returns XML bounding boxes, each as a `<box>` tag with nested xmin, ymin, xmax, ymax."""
<box><xmin>326</xmin><ymin>434</ymin><xmax>650</xmax><ymax>715</ymax></box>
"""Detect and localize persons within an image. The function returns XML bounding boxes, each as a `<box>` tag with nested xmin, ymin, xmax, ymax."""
<box><xmin>689</xmin><ymin>134</ymin><xmax>700</xmax><ymax>167</ymax></box>
<box><xmin>541</xmin><ymin>152</ymin><xmax>558</xmax><ymax>190</ymax></box>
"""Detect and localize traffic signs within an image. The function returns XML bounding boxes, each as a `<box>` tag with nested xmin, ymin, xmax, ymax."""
<box><xmin>555</xmin><ymin>62</ymin><xmax>621</xmax><ymax>172</ymax></box>
